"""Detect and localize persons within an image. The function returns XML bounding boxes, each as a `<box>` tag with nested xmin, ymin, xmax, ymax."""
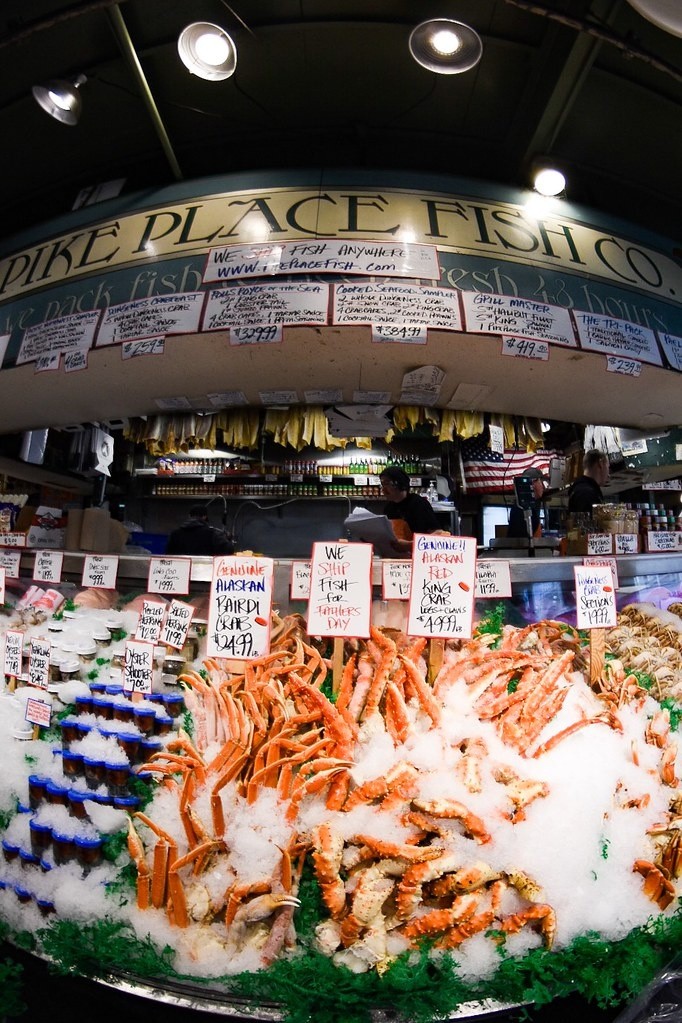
<box><xmin>362</xmin><ymin>466</ymin><xmax>443</xmax><ymax>558</ymax></box>
<box><xmin>507</xmin><ymin>468</ymin><xmax>549</xmax><ymax>538</ymax></box>
<box><xmin>166</xmin><ymin>506</ymin><xmax>234</xmax><ymax>555</ymax></box>
<box><xmin>567</xmin><ymin>449</ymin><xmax>609</xmax><ymax>519</ymax></box>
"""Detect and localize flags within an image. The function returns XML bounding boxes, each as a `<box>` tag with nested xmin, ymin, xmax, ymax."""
<box><xmin>458</xmin><ymin>423</ymin><xmax>557</xmax><ymax>493</ymax></box>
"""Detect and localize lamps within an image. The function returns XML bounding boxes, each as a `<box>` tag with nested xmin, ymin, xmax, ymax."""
<box><xmin>408</xmin><ymin>17</ymin><xmax>482</xmax><ymax>74</ymax></box>
<box><xmin>178</xmin><ymin>21</ymin><xmax>237</xmax><ymax>82</ymax></box>
<box><xmin>32</xmin><ymin>72</ymin><xmax>86</xmax><ymax>125</ymax></box>
<box><xmin>530</xmin><ymin>156</ymin><xmax>566</xmax><ymax>197</ymax></box>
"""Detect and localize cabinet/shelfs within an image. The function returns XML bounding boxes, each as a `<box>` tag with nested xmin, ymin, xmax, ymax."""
<box><xmin>133</xmin><ymin>471</ymin><xmax>435</xmax><ymax>501</ymax></box>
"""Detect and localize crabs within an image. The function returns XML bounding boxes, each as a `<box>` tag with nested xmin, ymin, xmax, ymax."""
<box><xmin>606</xmin><ymin>602</ymin><xmax>682</xmax><ymax>698</ymax></box>
<box><xmin>126</xmin><ymin>609</ymin><xmax>682</xmax><ymax>949</ymax></box>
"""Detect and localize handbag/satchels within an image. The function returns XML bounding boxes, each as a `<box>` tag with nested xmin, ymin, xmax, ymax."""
<box><xmin>26</xmin><ymin>506</ymin><xmax>63</xmax><ymax>549</ymax></box>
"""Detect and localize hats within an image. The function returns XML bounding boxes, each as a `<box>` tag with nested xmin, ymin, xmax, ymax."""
<box><xmin>523</xmin><ymin>468</ymin><xmax>549</xmax><ymax>478</ymax></box>
